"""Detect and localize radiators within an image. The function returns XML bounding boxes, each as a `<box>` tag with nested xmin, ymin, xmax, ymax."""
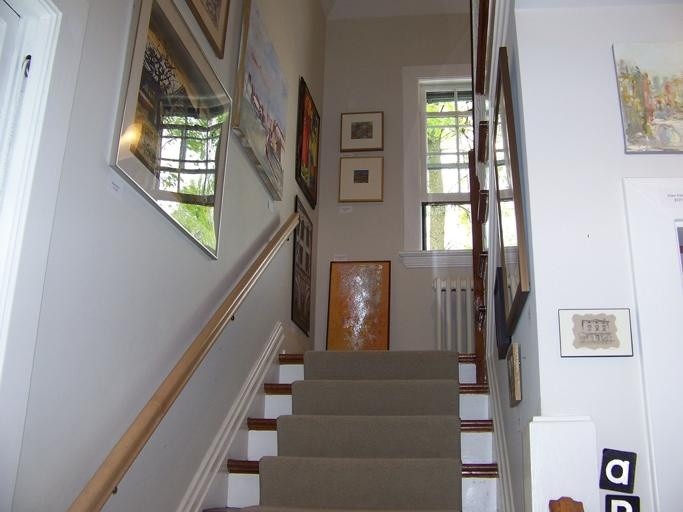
<box><xmin>431</xmin><ymin>274</ymin><xmax>475</xmax><ymax>354</ymax></box>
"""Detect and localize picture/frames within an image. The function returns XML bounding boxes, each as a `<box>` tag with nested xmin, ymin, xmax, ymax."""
<box><xmin>338</xmin><ymin>156</ymin><xmax>384</xmax><ymax>203</ymax></box>
<box><xmin>109</xmin><ymin>0</ymin><xmax>233</xmax><ymax>261</ymax></box>
<box><xmin>473</xmin><ymin>0</ymin><xmax>532</xmax><ymax>409</ymax></box>
<box><xmin>291</xmin><ymin>194</ymin><xmax>313</xmax><ymax>338</ymax></box>
<box><xmin>339</xmin><ymin>110</ymin><xmax>385</xmax><ymax>152</ymax></box>
<box><xmin>326</xmin><ymin>261</ymin><xmax>391</xmax><ymax>352</ymax></box>
<box><xmin>185</xmin><ymin>0</ymin><xmax>230</xmax><ymax>59</ymax></box>
